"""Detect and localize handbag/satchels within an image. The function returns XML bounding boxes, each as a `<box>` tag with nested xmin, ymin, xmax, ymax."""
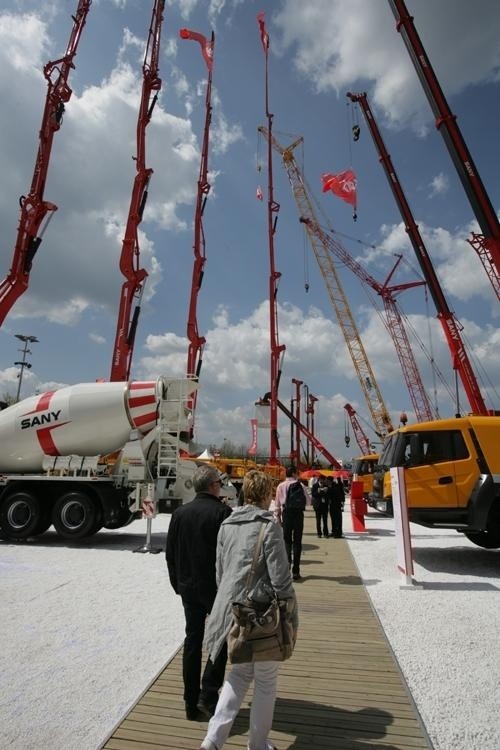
<box><xmin>227</xmin><ymin>597</ymin><xmax>298</xmax><ymax>664</ymax></box>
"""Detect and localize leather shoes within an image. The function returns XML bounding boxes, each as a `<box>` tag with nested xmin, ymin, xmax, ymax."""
<box><xmin>293</xmin><ymin>572</ymin><xmax>302</xmax><ymax>580</ymax></box>
<box><xmin>186</xmin><ymin>699</ymin><xmax>197</xmax><ymax>721</ymax></box>
<box><xmin>197</xmin><ymin>689</ymin><xmax>218</xmax><ymax>719</ymax></box>
<box><xmin>317</xmin><ymin>533</ymin><xmax>345</xmax><ymax>539</ymax></box>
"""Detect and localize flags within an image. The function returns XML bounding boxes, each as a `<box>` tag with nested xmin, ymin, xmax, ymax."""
<box><xmin>321</xmin><ymin>168</ymin><xmax>359</xmax><ymax>210</ymax></box>
<box><xmin>179</xmin><ymin>27</ymin><xmax>215</xmax><ymax>72</ymax></box>
<box><xmin>257</xmin><ymin>9</ymin><xmax>267</xmax><ymax>53</ymax></box>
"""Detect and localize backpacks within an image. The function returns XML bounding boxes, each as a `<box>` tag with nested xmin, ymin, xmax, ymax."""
<box><xmin>285</xmin><ymin>480</ymin><xmax>306</xmax><ymax>514</ymax></box>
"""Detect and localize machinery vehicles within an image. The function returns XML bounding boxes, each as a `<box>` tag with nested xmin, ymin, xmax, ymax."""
<box><xmin>368</xmin><ymin>416</ymin><xmax>500</xmax><ymax>549</ymax></box>
<box><xmin>0</xmin><ymin>373</ymin><xmax>202</xmax><ymax>554</ymax></box>
<box><xmin>351</xmin><ymin>453</ymin><xmax>379</xmax><ymax>496</ymax></box>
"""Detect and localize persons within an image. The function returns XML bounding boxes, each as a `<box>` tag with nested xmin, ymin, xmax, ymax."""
<box><xmin>311</xmin><ymin>475</ymin><xmax>329</xmax><ymax>537</ymax></box>
<box><xmin>323</xmin><ymin>476</ymin><xmax>346</xmax><ymax>539</ymax></box>
<box><xmin>165</xmin><ymin>467</ymin><xmax>236</xmax><ymax>723</ymax></box>
<box><xmin>198</xmin><ymin>470</ymin><xmax>299</xmax><ymax>750</ymax></box>
<box><xmin>274</xmin><ymin>466</ymin><xmax>313</xmax><ymax>581</ymax></box>
<box><xmin>337</xmin><ymin>476</ymin><xmax>345</xmax><ymax>511</ymax></box>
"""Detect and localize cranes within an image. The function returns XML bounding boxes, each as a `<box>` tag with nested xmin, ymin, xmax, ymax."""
<box><xmin>298</xmin><ymin>215</ymin><xmax>439</xmax><ymax>422</ymax></box>
<box><xmin>256</xmin><ymin>124</ymin><xmax>395</xmax><ymax>446</ymax></box>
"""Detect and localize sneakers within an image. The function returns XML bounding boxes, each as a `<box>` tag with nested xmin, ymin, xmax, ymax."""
<box><xmin>268</xmin><ymin>743</ymin><xmax>278</xmax><ymax>750</ymax></box>
<box><xmin>199</xmin><ymin>735</ymin><xmax>223</xmax><ymax>750</ymax></box>
